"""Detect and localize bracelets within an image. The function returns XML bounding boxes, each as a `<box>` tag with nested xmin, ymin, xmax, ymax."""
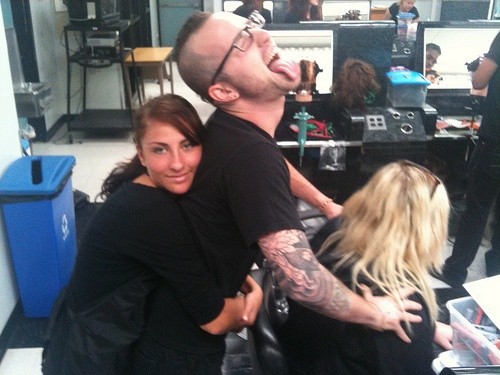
<box><xmin>319</xmin><ymin>198</ymin><xmax>333</xmax><ymax>212</ymax></box>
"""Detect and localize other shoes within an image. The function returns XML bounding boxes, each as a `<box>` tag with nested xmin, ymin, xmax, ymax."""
<box><xmin>430</xmin><ymin>258</ymin><xmax>446</xmax><ymax>279</ymax></box>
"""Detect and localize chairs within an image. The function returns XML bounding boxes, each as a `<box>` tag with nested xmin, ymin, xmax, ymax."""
<box><xmin>250</xmin><ymin>266</ymin><xmax>292</xmax><ymax>375</ymax></box>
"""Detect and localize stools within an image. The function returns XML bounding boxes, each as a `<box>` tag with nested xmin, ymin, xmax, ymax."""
<box><xmin>124</xmin><ymin>46</ymin><xmax>174</xmax><ymax>97</ymax></box>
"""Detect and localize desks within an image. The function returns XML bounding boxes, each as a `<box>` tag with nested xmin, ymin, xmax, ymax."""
<box><xmin>274</xmin><ymin>88</ymin><xmax>487</xmax><ymax>238</ymax></box>
<box><xmin>370</xmin><ymin>5</ymin><xmax>389</xmax><ymax>20</ymax></box>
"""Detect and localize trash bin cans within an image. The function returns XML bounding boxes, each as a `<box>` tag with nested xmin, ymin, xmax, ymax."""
<box><xmin>1</xmin><ymin>155</ymin><xmax>77</xmax><ymax>319</ymax></box>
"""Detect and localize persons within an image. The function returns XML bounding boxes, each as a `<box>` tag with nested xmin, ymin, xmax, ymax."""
<box><xmin>426</xmin><ymin>32</ymin><xmax>500</xmax><ymax>288</ymax></box>
<box><xmin>127</xmin><ymin>12</ymin><xmax>422</xmax><ymax>375</ymax></box>
<box><xmin>232</xmin><ymin>0</ymin><xmax>441</xmax><ymax>126</ymax></box>
<box><xmin>287</xmin><ymin>160</ymin><xmax>454</xmax><ymax>375</ymax></box>
<box><xmin>41</xmin><ymin>94</ymin><xmax>262</xmax><ymax>375</ymax></box>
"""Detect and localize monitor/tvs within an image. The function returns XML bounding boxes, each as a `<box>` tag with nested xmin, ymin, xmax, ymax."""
<box><xmin>222</xmin><ymin>0</ymin><xmax>500</xmax><ymax>102</ymax></box>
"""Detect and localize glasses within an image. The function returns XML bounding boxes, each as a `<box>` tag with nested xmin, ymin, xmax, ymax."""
<box><xmin>426</xmin><ymin>52</ymin><xmax>437</xmax><ymax>65</ymax></box>
<box><xmin>212</xmin><ymin>10</ymin><xmax>266</xmax><ymax>84</ymax></box>
<box><xmin>407</xmin><ymin>159</ymin><xmax>440</xmax><ymax>202</ymax></box>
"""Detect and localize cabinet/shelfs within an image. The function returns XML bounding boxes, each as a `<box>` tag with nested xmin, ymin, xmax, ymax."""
<box><xmin>63</xmin><ymin>13</ymin><xmax>152</xmax><ymax>146</ymax></box>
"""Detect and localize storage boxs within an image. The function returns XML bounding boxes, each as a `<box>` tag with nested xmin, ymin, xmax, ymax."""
<box><xmin>397</xmin><ymin>25</ymin><xmax>408</xmax><ymax>36</ymax></box>
<box><xmin>386</xmin><ymin>70</ymin><xmax>431</xmax><ymax>111</ymax></box>
<box><xmin>396</xmin><ymin>12</ymin><xmax>417</xmax><ymax>25</ymax></box>
<box><xmin>446</xmin><ymin>294</ymin><xmax>500</xmax><ymax>368</ymax></box>
